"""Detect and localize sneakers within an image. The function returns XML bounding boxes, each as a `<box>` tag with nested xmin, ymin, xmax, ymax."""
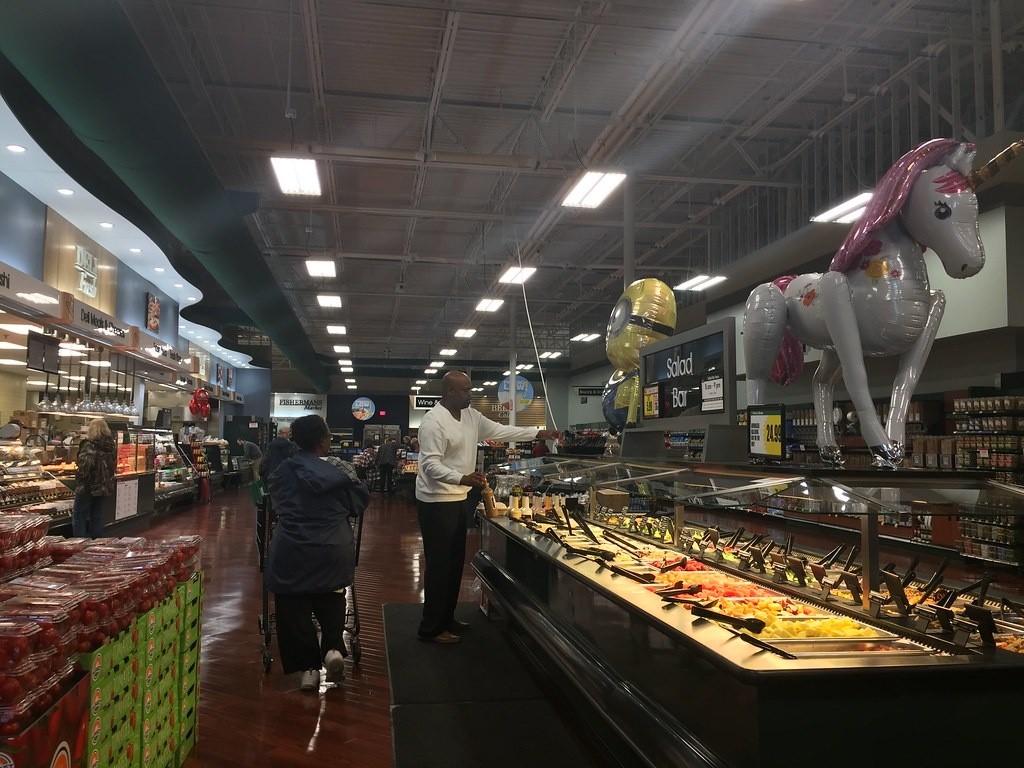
<box><xmin>299</xmin><ymin>670</ymin><xmax>322</xmax><ymax>693</ymax></box>
<box><xmin>325</xmin><ymin>649</ymin><xmax>346</xmax><ymax>683</ymax></box>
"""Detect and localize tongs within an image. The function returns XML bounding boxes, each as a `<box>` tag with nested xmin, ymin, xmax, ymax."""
<box><xmin>506</xmin><ymin>503</ymin><xmax>1024</xmax><ymax>635</ymax></box>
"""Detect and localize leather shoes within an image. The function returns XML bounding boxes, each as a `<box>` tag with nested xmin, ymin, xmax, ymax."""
<box><xmin>418</xmin><ymin>629</ymin><xmax>463</xmax><ymax>644</ymax></box>
<box><xmin>440</xmin><ymin>619</ymin><xmax>473</xmax><ymax>631</ymax></box>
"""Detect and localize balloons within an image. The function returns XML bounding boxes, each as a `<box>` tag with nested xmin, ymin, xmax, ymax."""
<box><xmin>189</xmin><ymin>387</ymin><xmax>211</xmax><ymax>417</ymax></box>
<box><xmin>739</xmin><ymin>138</ymin><xmax>1024</xmax><ymax>467</ymax></box>
<box><xmin>597</xmin><ymin>277</ymin><xmax>678</xmax><ymax>446</ymax></box>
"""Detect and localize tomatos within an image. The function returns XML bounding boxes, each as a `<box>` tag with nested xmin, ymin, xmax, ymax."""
<box><xmin>0</xmin><ymin>511</ymin><xmax>201</xmax><ymax>737</ymax></box>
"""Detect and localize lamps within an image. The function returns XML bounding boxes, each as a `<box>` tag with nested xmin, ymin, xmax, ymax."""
<box><xmin>37</xmin><ymin>322</ymin><xmax>141</xmax><ymax>417</ymax></box>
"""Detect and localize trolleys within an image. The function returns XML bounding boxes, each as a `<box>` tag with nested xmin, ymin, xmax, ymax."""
<box><xmin>256</xmin><ymin>492</ymin><xmax>366</xmax><ymax>672</ymax></box>
<box><xmin>366</xmin><ymin>462</ymin><xmax>389</xmax><ymax>493</ymax></box>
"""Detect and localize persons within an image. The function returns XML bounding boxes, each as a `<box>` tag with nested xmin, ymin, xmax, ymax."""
<box><xmin>270</xmin><ymin>427</ymin><xmax>293</xmax><ymax>472</ymax></box>
<box><xmin>237</xmin><ymin>438</ymin><xmax>262</xmax><ymax>481</ymax></box>
<box><xmin>266</xmin><ymin>415</ymin><xmax>370</xmax><ymax>693</ymax></box>
<box><xmin>418</xmin><ymin>370</ymin><xmax>562</xmax><ymax>647</ymax></box>
<box><xmin>360</xmin><ymin>436</ymin><xmax>419</xmax><ymax>514</ymax></box>
<box><xmin>52</xmin><ymin>432</ymin><xmax>64</xmax><ymax>444</ymax></box>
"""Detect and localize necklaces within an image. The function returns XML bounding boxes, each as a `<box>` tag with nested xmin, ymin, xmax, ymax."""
<box><xmin>72</xmin><ymin>417</ymin><xmax>117</xmax><ymax>537</ymax></box>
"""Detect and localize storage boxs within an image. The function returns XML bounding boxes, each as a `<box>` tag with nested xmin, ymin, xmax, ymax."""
<box><xmin>75</xmin><ymin>567</ymin><xmax>206</xmax><ymax>767</ymax></box>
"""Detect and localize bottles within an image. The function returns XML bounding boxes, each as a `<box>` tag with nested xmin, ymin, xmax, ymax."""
<box><xmin>480</xmin><ymin>475</ymin><xmax>571</xmax><ymax>522</ymax></box>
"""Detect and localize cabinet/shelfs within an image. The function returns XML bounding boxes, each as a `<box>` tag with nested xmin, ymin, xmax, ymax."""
<box><xmin>0</xmin><ymin>409</ymin><xmax>280</xmax><ymax>533</ymax></box>
<box><xmin>476</xmin><ymin>380</ymin><xmax>1024</xmax><ymax>768</ymax></box>
